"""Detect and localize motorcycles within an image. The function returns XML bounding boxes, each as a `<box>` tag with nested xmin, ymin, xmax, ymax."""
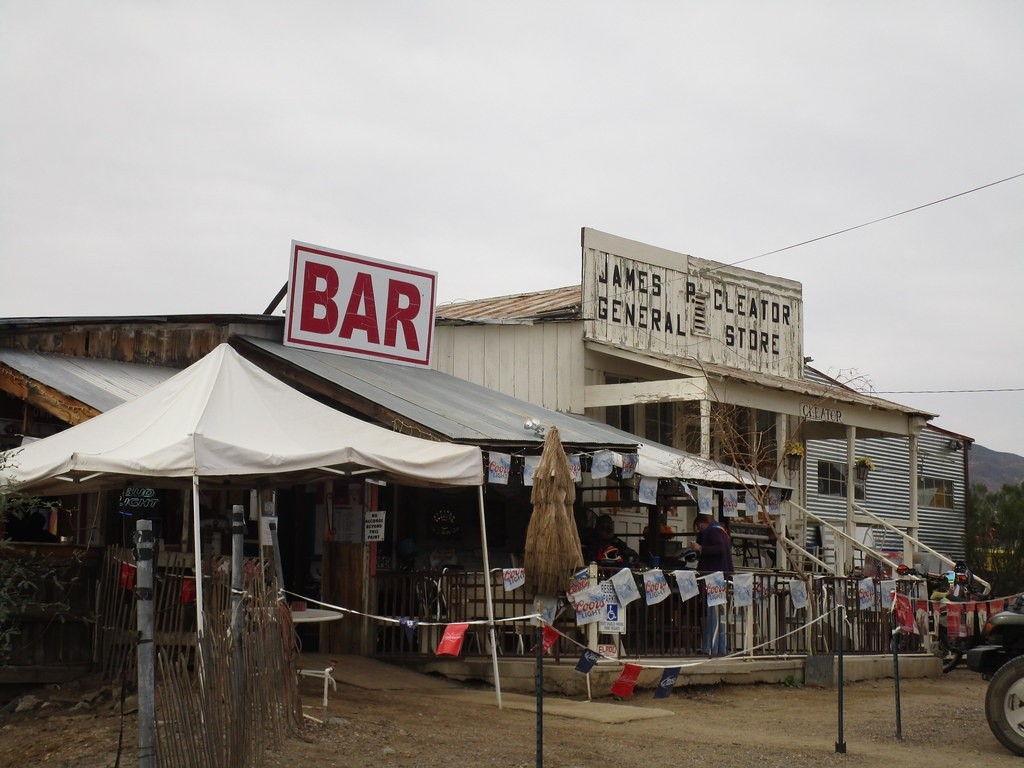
<box><xmin>895</xmin><ymin>564</ymin><xmax>968</xmax><ymax>673</ymax></box>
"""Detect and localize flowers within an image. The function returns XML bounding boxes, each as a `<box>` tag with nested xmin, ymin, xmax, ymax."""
<box><xmin>856</xmin><ymin>456</ymin><xmax>874</xmax><ymax>471</ymax></box>
<box><xmin>785</xmin><ymin>439</ymin><xmax>803</xmax><ymax>456</ymax></box>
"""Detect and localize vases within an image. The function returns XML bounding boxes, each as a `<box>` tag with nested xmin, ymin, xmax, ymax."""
<box><xmin>856</xmin><ymin>467</ymin><xmax>869</xmax><ymax>479</ymax></box>
<box><xmin>783</xmin><ymin>454</ymin><xmax>801</xmax><ymax>481</ymax></box>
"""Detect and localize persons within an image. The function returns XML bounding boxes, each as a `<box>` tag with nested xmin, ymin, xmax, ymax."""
<box><xmin>586</xmin><ymin>512</ymin><xmax>637</xmax><ymax>577</ymax></box>
<box><xmin>693</xmin><ymin>512</ymin><xmax>735</xmax><ymax>657</ymax></box>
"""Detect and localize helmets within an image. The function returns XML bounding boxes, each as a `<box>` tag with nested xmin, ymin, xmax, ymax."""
<box><xmin>952</xmin><ymin>560</ymin><xmax>971</xmax><ymax>584</ymax></box>
<box><xmin>594</xmin><ymin>544</ymin><xmax>623</xmax><ymax>566</ymax></box>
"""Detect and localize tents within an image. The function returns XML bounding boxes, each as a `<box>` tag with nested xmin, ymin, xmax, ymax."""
<box><xmin>0</xmin><ymin>342</ymin><xmax>485</xmax><ymax>494</ymax></box>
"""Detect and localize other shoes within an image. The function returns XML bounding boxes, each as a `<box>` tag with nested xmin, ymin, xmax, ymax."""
<box><xmin>697</xmin><ymin>650</ymin><xmax>711</xmax><ymax>657</ymax></box>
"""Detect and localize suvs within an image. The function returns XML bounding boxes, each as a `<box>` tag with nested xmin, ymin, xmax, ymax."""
<box><xmin>965</xmin><ymin>592</ymin><xmax>1024</xmax><ymax>756</ymax></box>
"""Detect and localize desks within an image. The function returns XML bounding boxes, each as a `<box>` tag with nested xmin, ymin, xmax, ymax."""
<box><xmin>222</xmin><ymin>608</ymin><xmax>343</xmax><ymax>723</ymax></box>
